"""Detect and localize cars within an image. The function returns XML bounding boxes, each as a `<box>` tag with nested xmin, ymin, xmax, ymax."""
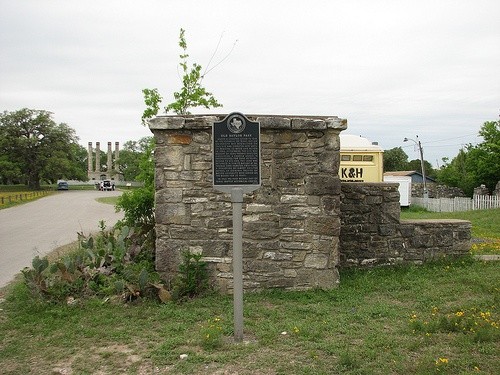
<box><xmin>58</xmin><ymin>182</ymin><xmax>68</xmax><ymax>190</ymax></box>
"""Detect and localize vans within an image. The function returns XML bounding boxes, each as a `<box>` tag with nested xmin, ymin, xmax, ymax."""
<box><xmin>100</xmin><ymin>181</ymin><xmax>113</xmax><ymax>191</ymax></box>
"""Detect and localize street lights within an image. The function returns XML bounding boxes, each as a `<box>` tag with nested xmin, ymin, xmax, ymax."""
<box><xmin>403</xmin><ymin>135</ymin><xmax>427</xmax><ymax>197</ymax></box>
